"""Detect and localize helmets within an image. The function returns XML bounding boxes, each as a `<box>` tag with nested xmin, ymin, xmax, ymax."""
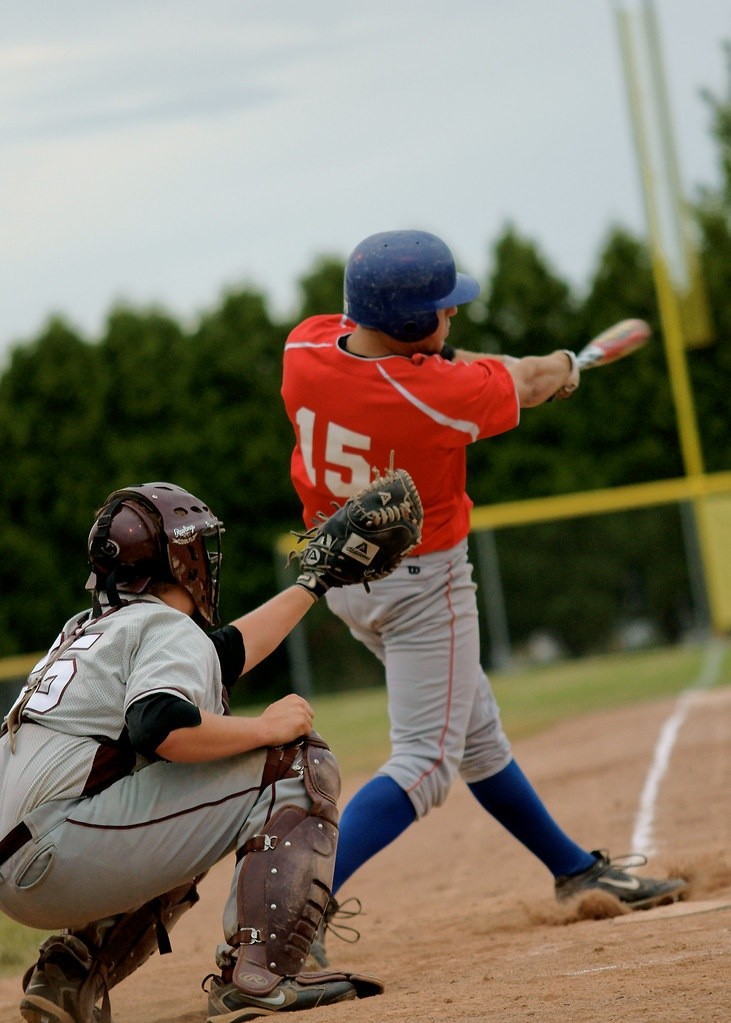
<box><xmin>344</xmin><ymin>230</ymin><xmax>479</xmax><ymax>342</ymax></box>
<box><xmin>85</xmin><ymin>480</ymin><xmax>225</xmax><ymax>628</ymax></box>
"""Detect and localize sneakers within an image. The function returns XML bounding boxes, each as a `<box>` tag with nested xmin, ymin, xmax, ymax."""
<box><xmin>555</xmin><ymin>850</ymin><xmax>686</xmax><ymax>912</ymax></box>
<box><xmin>20</xmin><ymin>958</ymin><xmax>84</xmax><ymax>1023</ymax></box>
<box><xmin>297</xmin><ymin>895</ymin><xmax>365</xmax><ymax>973</ymax></box>
<box><xmin>201</xmin><ymin>968</ymin><xmax>356</xmax><ymax>1023</ymax></box>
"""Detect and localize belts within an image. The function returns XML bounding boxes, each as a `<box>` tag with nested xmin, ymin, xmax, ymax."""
<box><xmin>0</xmin><ymin>821</ymin><xmax>32</xmax><ymax>866</ymax></box>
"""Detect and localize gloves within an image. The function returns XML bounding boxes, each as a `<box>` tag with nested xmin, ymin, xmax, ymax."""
<box><xmin>554</xmin><ymin>349</ymin><xmax>582</xmax><ymax>399</ymax></box>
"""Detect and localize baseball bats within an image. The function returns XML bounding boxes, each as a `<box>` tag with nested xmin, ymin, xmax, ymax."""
<box><xmin>546</xmin><ymin>317</ymin><xmax>653</xmax><ymax>404</ymax></box>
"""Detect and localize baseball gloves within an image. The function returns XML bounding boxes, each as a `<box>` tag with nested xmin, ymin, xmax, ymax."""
<box><xmin>285</xmin><ymin>446</ymin><xmax>425</xmax><ymax>605</ymax></box>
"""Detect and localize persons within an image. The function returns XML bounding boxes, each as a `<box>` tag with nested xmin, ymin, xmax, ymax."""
<box><xmin>0</xmin><ymin>449</ymin><xmax>422</xmax><ymax>1023</ymax></box>
<box><xmin>262</xmin><ymin>232</ymin><xmax>691</xmax><ymax>979</ymax></box>
